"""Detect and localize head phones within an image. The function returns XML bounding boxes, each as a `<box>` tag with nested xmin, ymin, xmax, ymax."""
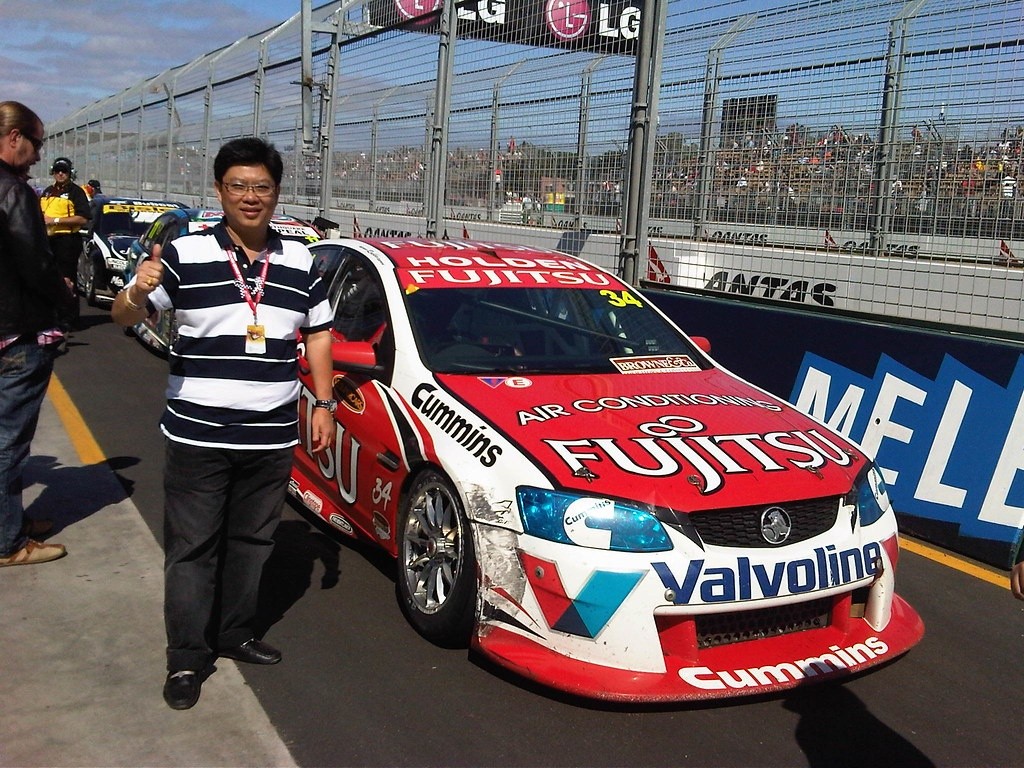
<box><xmin>87</xmin><ymin>181</ymin><xmax>96</xmax><ymax>194</ymax></box>
<box><xmin>50</xmin><ymin>158</ymin><xmax>77</xmax><ymax>182</ymax></box>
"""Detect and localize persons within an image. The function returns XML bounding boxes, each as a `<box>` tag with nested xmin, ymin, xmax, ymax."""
<box><xmin>602</xmin><ymin>179</ymin><xmax>622</xmax><ymax>192</ymax></box>
<box><xmin>40</xmin><ymin>157</ymin><xmax>91</xmax><ymax>325</ymax></box>
<box><xmin>110</xmin><ymin>138</ymin><xmax>334</xmax><ymax>709</ymax></box>
<box><xmin>88</xmin><ymin>180</ymin><xmax>110</xmax><ymax>238</ymax></box>
<box><xmin>654</xmin><ymin>123</ymin><xmax>1024</xmax><ymax>218</ymax></box>
<box><xmin>35</xmin><ymin>186</ymin><xmax>42</xmax><ymax>196</ymax></box>
<box><xmin>0</xmin><ymin>101</ymin><xmax>65</xmax><ymax>568</ymax></box>
<box><xmin>333</xmin><ymin>135</ymin><xmax>542</xmax><ymax>210</ymax></box>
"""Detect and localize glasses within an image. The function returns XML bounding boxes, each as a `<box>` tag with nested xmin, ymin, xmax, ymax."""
<box><xmin>18</xmin><ymin>129</ymin><xmax>43</xmax><ymax>152</ymax></box>
<box><xmin>221</xmin><ymin>180</ymin><xmax>277</xmax><ymax>197</ymax></box>
<box><xmin>54</xmin><ymin>166</ymin><xmax>69</xmax><ymax>174</ymax></box>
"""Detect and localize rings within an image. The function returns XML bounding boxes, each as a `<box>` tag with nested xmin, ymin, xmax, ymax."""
<box><xmin>147</xmin><ymin>277</ymin><xmax>152</xmax><ymax>284</ymax></box>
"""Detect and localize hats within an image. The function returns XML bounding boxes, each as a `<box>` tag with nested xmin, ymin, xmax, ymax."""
<box><xmin>53</xmin><ymin>157</ymin><xmax>72</xmax><ymax>170</ymax></box>
<box><xmin>88</xmin><ymin>180</ymin><xmax>102</xmax><ymax>194</ymax></box>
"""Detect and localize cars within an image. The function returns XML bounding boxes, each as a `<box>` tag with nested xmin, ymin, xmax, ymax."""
<box><xmin>121</xmin><ymin>206</ymin><xmax>323</xmax><ymax>359</ymax></box>
<box><xmin>283</xmin><ymin>237</ymin><xmax>925</xmax><ymax>705</ymax></box>
<box><xmin>71</xmin><ymin>191</ymin><xmax>193</xmax><ymax>304</ymax></box>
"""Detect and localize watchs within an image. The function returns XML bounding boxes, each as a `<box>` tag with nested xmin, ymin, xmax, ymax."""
<box><xmin>316</xmin><ymin>400</ymin><xmax>337</xmax><ymax>411</ymax></box>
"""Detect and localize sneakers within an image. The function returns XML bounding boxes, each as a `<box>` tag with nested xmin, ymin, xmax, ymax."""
<box><xmin>0</xmin><ymin>537</ymin><xmax>66</xmax><ymax>567</ymax></box>
<box><xmin>21</xmin><ymin>518</ymin><xmax>54</xmax><ymax>536</ymax></box>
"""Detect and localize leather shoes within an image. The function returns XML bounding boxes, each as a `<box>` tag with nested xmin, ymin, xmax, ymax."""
<box><xmin>163</xmin><ymin>667</ymin><xmax>200</xmax><ymax>710</ymax></box>
<box><xmin>217</xmin><ymin>637</ymin><xmax>282</xmax><ymax>665</ymax></box>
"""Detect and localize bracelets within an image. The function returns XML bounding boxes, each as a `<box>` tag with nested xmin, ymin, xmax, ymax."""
<box><xmin>54</xmin><ymin>217</ymin><xmax>59</xmax><ymax>225</ymax></box>
<box><xmin>124</xmin><ymin>288</ymin><xmax>144</xmax><ymax>312</ymax></box>
